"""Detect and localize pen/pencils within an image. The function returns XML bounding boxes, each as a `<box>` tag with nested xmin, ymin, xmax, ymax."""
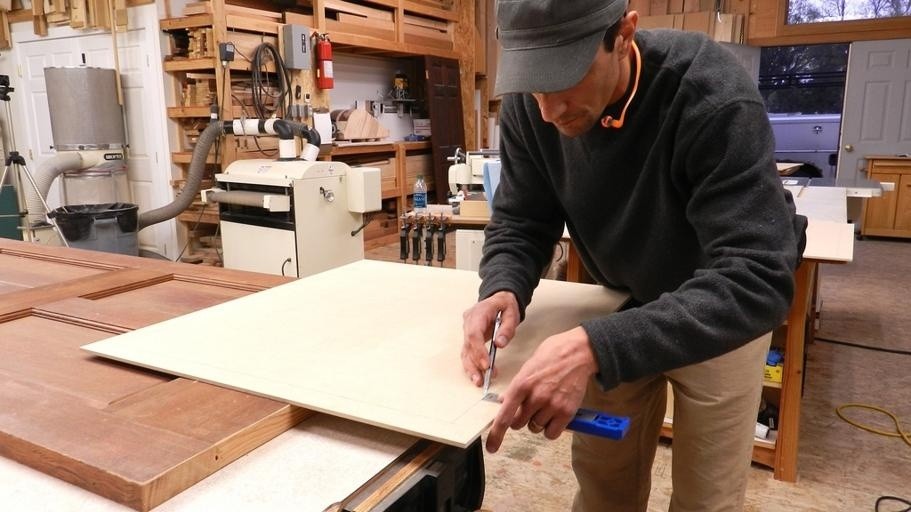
<box><xmin>482</xmin><ymin>311</ymin><xmax>501</xmax><ymax>397</ymax></box>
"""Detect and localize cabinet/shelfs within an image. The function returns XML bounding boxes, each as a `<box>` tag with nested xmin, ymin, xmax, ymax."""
<box><xmin>856</xmin><ymin>155</ymin><xmax>911</xmax><ymax>240</ymax></box>
<box><xmin>159</xmin><ymin>1</ymin><xmax>284</xmax><ymax>267</ymax></box>
<box><xmin>331</xmin><ymin>140</ymin><xmax>433</xmax><ymax>252</ymax></box>
<box><xmin>284</xmin><ymin>1</ymin><xmax>458</xmax><ymax>54</ymax></box>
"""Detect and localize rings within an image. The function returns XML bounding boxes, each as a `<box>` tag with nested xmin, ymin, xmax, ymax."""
<box><xmin>529</xmin><ymin>416</ymin><xmax>547</xmax><ymax>431</ymax></box>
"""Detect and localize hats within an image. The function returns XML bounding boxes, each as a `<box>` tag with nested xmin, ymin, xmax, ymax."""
<box><xmin>493</xmin><ymin>0</ymin><xmax>629</xmax><ymax>100</ymax></box>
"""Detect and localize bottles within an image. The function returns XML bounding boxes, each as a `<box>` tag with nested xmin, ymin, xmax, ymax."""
<box><xmin>413</xmin><ymin>174</ymin><xmax>428</xmax><ymax>213</ymax></box>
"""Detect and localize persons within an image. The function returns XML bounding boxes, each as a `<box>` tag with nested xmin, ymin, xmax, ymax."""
<box><xmin>458</xmin><ymin>0</ymin><xmax>808</xmax><ymax>512</ymax></box>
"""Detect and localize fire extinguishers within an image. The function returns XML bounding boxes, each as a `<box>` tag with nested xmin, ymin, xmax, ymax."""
<box><xmin>311</xmin><ymin>31</ymin><xmax>334</xmax><ymax>90</ymax></box>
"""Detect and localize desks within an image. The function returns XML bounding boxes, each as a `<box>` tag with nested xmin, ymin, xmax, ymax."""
<box><xmin>1</xmin><ymin>413</ymin><xmax>486</xmax><ymax>512</ymax></box>
<box><xmin>399</xmin><ymin>200</ymin><xmax>854</xmax><ymax>484</ymax></box>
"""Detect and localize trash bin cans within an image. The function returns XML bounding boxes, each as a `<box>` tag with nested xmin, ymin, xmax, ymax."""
<box><xmin>49</xmin><ymin>204</ymin><xmax>140</xmax><ymax>257</ymax></box>
